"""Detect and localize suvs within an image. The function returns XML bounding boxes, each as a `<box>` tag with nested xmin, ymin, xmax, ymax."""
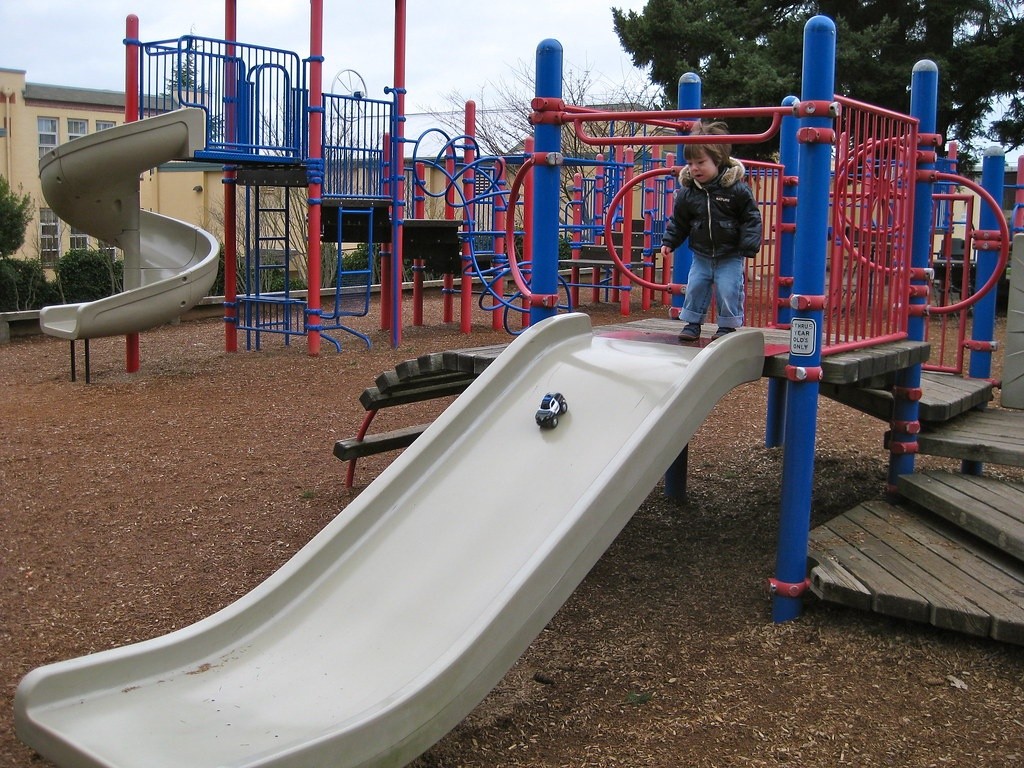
<box><xmin>534</xmin><ymin>391</ymin><xmax>568</xmax><ymax>429</ymax></box>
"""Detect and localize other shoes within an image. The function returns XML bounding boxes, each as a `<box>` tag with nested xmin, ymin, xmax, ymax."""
<box><xmin>678</xmin><ymin>324</ymin><xmax>701</xmax><ymax>340</ymax></box>
<box><xmin>712</xmin><ymin>327</ymin><xmax>736</xmax><ymax>340</ymax></box>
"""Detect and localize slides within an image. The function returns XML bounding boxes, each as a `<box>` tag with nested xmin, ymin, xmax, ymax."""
<box><xmin>38</xmin><ymin>109</ymin><xmax>221</xmax><ymax>341</ymax></box>
<box><xmin>11</xmin><ymin>309</ymin><xmax>767</xmax><ymax>768</ymax></box>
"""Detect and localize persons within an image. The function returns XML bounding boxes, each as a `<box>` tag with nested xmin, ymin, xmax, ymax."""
<box><xmin>660</xmin><ymin>121</ymin><xmax>761</xmax><ymax>339</ymax></box>
